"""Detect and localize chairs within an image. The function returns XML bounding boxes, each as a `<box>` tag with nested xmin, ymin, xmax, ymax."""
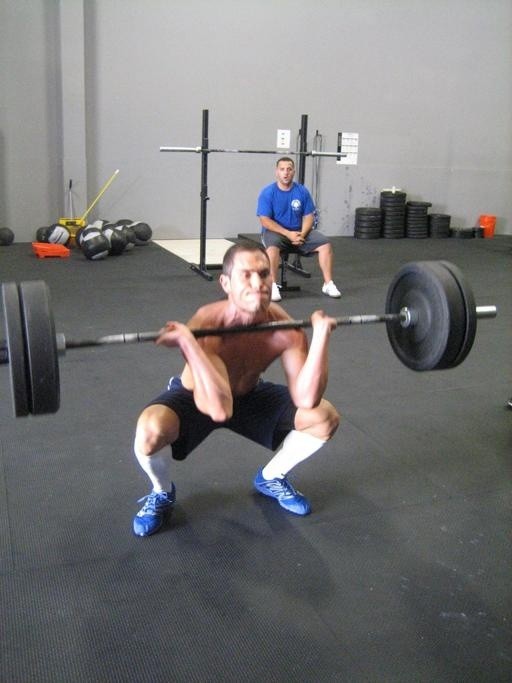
<box><xmin>254</xmin><ymin>464</ymin><xmax>311</xmax><ymax>516</ymax></box>
<box><xmin>322</xmin><ymin>280</ymin><xmax>341</xmax><ymax>298</ymax></box>
<box><xmin>271</xmin><ymin>282</ymin><xmax>282</xmax><ymax>301</ymax></box>
<box><xmin>133</xmin><ymin>481</ymin><xmax>175</xmax><ymax>536</ymax></box>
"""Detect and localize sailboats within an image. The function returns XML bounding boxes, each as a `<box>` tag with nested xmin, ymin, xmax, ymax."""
<box><xmin>0</xmin><ymin>220</ymin><xmax>152</xmax><ymax>261</ymax></box>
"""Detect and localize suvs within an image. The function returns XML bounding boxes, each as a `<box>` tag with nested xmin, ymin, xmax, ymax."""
<box><xmin>480</xmin><ymin>215</ymin><xmax>496</xmax><ymax>237</ymax></box>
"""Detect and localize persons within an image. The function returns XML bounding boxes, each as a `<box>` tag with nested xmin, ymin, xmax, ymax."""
<box><xmin>130</xmin><ymin>239</ymin><xmax>341</xmax><ymax>536</ymax></box>
<box><xmin>256</xmin><ymin>157</ymin><xmax>341</xmax><ymax>301</ymax></box>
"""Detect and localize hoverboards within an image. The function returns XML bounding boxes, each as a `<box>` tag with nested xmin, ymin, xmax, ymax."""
<box><xmin>2</xmin><ymin>262</ymin><xmax>498</xmax><ymax>417</ymax></box>
